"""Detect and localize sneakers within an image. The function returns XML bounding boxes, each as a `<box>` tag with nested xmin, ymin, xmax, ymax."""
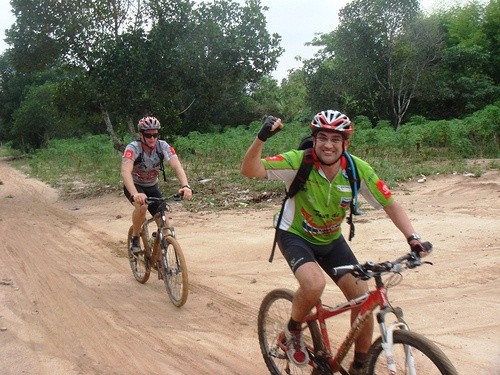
<box><xmin>277</xmin><ymin>327</ymin><xmax>309</xmax><ymax>368</ymax></box>
<box><xmin>348</xmin><ymin>362</ymin><xmax>378</xmax><ymax>375</ymax></box>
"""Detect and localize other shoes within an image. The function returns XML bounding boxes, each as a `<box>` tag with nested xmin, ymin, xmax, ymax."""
<box><xmin>131</xmin><ymin>236</ymin><xmax>143</xmax><ymax>254</ymax></box>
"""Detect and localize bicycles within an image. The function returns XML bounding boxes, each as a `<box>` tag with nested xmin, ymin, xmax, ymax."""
<box><xmin>128</xmin><ymin>193</ymin><xmax>189</xmax><ymax>307</ymax></box>
<box><xmin>258</xmin><ymin>249</ymin><xmax>458</xmax><ymax>375</ymax></box>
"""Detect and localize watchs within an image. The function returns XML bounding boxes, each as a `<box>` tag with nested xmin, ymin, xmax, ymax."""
<box><xmin>408</xmin><ymin>234</ymin><xmax>421</xmax><ymax>245</ymax></box>
<box><xmin>183</xmin><ymin>185</ymin><xmax>190</xmax><ymax>189</ymax></box>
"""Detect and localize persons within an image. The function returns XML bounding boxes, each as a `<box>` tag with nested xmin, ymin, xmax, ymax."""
<box><xmin>121</xmin><ymin>117</ymin><xmax>192</xmax><ymax>271</ymax></box>
<box><xmin>241</xmin><ymin>110</ymin><xmax>433</xmax><ymax>375</ymax></box>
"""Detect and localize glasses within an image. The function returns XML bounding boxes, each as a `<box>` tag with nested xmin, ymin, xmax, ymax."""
<box><xmin>140</xmin><ymin>130</ymin><xmax>159</xmax><ymax>138</ymax></box>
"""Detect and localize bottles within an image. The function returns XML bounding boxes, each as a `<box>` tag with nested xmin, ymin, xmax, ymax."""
<box><xmin>148</xmin><ymin>231</ymin><xmax>156</xmax><ymax>248</ymax></box>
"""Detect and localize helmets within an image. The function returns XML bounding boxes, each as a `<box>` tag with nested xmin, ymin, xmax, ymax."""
<box><xmin>310</xmin><ymin>110</ymin><xmax>353</xmax><ymax>135</ymax></box>
<box><xmin>138</xmin><ymin>115</ymin><xmax>161</xmax><ymax>131</ymax></box>
